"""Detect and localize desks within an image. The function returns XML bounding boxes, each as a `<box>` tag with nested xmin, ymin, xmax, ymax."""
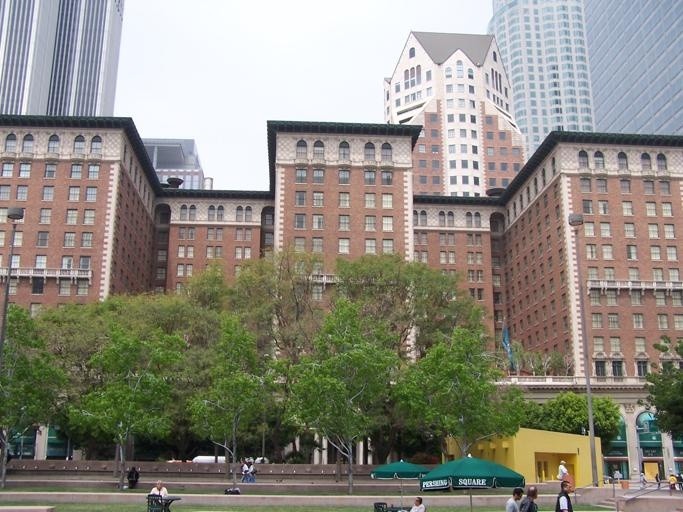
<box><xmin>388</xmin><ymin>507</ymin><xmax>412</xmax><ymax>512</ymax></box>
<box><xmin>146</xmin><ymin>496</ymin><xmax>182</xmax><ymax>512</ymax></box>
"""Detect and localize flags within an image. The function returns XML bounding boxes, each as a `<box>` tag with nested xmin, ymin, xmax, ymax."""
<box><xmin>500</xmin><ymin>323</ymin><xmax>514</xmax><ymax>372</ymax></box>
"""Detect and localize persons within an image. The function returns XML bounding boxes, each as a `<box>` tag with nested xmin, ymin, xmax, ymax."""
<box><xmin>127</xmin><ymin>467</ymin><xmax>139</xmax><ymax>489</ymax></box>
<box><xmin>240</xmin><ymin>460</ymin><xmax>256</xmax><ymax>483</ymax></box>
<box><xmin>556</xmin><ymin>460</ymin><xmax>568</xmax><ymax>480</ymax></box>
<box><xmin>640</xmin><ymin>473</ymin><xmax>683</xmax><ymax>491</ymax></box>
<box><xmin>410</xmin><ymin>497</ymin><xmax>426</xmax><ymax>512</ymax></box>
<box><xmin>519</xmin><ymin>486</ymin><xmax>538</xmax><ymax>512</ymax></box>
<box><xmin>506</xmin><ymin>488</ymin><xmax>524</xmax><ymax>512</ymax></box>
<box><xmin>151</xmin><ymin>480</ymin><xmax>168</xmax><ymax>504</ymax></box>
<box><xmin>555</xmin><ymin>481</ymin><xmax>573</xmax><ymax>512</ymax></box>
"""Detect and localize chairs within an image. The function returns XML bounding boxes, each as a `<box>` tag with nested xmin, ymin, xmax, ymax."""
<box><xmin>374</xmin><ymin>502</ymin><xmax>387</xmax><ymax>512</ymax></box>
<box><xmin>148</xmin><ymin>494</ymin><xmax>163</xmax><ymax>512</ymax></box>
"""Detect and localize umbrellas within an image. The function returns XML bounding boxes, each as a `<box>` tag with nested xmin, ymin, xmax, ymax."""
<box><xmin>420</xmin><ymin>453</ymin><xmax>525</xmax><ymax>512</ymax></box>
<box><xmin>369</xmin><ymin>459</ymin><xmax>431</xmax><ymax>512</ymax></box>
